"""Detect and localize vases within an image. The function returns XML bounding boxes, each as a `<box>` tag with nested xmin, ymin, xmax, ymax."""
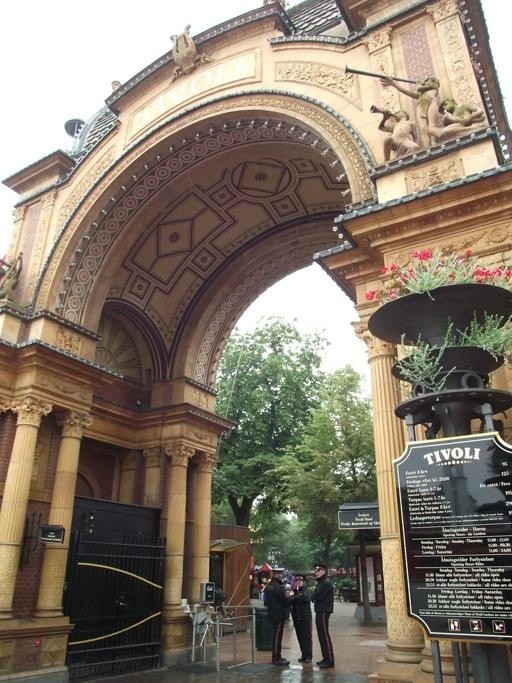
<box><xmin>368</xmin><ymin>283</ymin><xmax>512</xmax><ymax>349</ymax></box>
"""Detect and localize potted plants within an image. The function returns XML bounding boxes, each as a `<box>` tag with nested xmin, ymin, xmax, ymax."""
<box><xmin>391</xmin><ymin>309</ymin><xmax>512</xmax><ymax>440</ymax></box>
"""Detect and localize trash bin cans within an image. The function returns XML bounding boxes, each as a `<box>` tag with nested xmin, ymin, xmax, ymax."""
<box><xmin>254</xmin><ymin>607</ymin><xmax>275</xmax><ymax>651</ymax></box>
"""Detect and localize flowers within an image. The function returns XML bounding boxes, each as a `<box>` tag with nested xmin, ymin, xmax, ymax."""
<box><xmin>365</xmin><ymin>247</ymin><xmax>512</xmax><ymax>307</ymax></box>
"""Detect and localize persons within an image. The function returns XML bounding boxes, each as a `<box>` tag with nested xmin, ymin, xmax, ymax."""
<box><xmin>377</xmin><ymin>76</ymin><xmax>486</xmax><ymax>160</ymax></box>
<box><xmin>310</xmin><ymin>563</ymin><xmax>334</xmax><ymax>668</ymax></box>
<box><xmin>289</xmin><ymin>574</ymin><xmax>313</xmax><ymax>663</ymax></box>
<box><xmin>263</xmin><ymin>568</ymin><xmax>291</xmax><ymax>666</ymax></box>
<box><xmin>0</xmin><ymin>255</ymin><xmax>23</xmax><ymax>300</ymax></box>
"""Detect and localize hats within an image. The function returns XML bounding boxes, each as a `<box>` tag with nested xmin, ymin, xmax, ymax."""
<box><xmin>295</xmin><ymin>574</ymin><xmax>307</xmax><ymax>581</ymax></box>
<box><xmin>313</xmin><ymin>564</ymin><xmax>326</xmax><ymax>572</ymax></box>
<box><xmin>271</xmin><ymin>567</ymin><xmax>285</xmax><ymax>573</ymax></box>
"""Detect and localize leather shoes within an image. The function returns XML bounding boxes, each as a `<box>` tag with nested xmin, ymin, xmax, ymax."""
<box><xmin>272</xmin><ymin>655</ymin><xmax>335</xmax><ymax>669</ymax></box>
